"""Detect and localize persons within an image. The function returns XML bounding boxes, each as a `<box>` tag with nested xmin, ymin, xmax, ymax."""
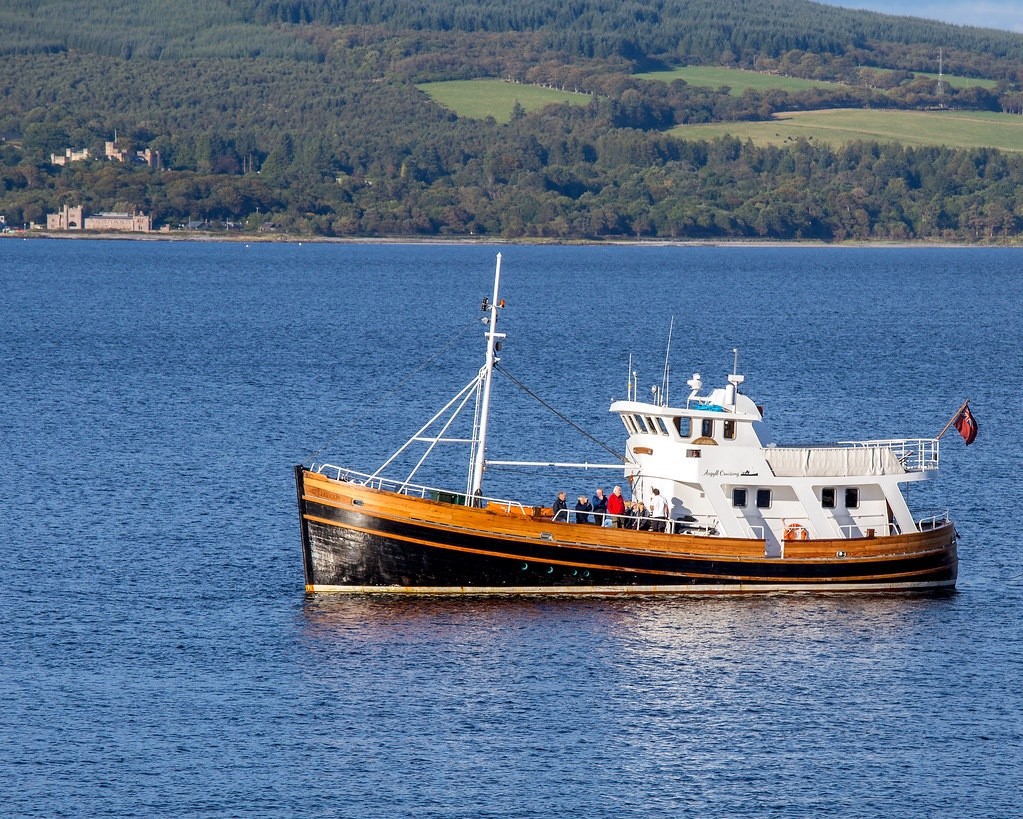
<box><xmin>650</xmin><ymin>489</ymin><xmax>669</xmax><ymax>533</ymax></box>
<box><xmin>592</xmin><ymin>488</ymin><xmax>608</xmax><ymax>526</ymax></box>
<box><xmin>607</xmin><ymin>486</ymin><xmax>626</xmax><ymax>529</ymax></box>
<box><xmin>575</xmin><ymin>495</ymin><xmax>592</xmax><ymax>523</ymax></box>
<box><xmin>623</xmin><ymin>499</ymin><xmax>651</xmax><ymax>530</ymax></box>
<box><xmin>552</xmin><ymin>491</ymin><xmax>571</xmax><ymax>523</ymax></box>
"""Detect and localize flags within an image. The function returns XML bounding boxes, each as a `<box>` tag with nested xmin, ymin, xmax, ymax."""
<box><xmin>952</xmin><ymin>399</ymin><xmax>978</xmax><ymax>446</ymax></box>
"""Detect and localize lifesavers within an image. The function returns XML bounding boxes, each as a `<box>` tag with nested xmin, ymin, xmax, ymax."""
<box><xmin>784</xmin><ymin>523</ymin><xmax>807</xmax><ymax>540</ymax></box>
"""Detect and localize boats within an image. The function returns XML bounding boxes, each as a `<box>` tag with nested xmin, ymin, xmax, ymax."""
<box><xmin>293</xmin><ymin>249</ymin><xmax>960</xmax><ymax>597</ymax></box>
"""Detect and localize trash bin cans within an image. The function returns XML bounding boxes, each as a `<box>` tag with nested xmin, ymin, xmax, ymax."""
<box><xmin>455</xmin><ymin>494</ymin><xmax>472</xmax><ymax>508</ymax></box>
<box><xmin>430</xmin><ymin>489</ymin><xmax>455</xmax><ymax>504</ymax></box>
